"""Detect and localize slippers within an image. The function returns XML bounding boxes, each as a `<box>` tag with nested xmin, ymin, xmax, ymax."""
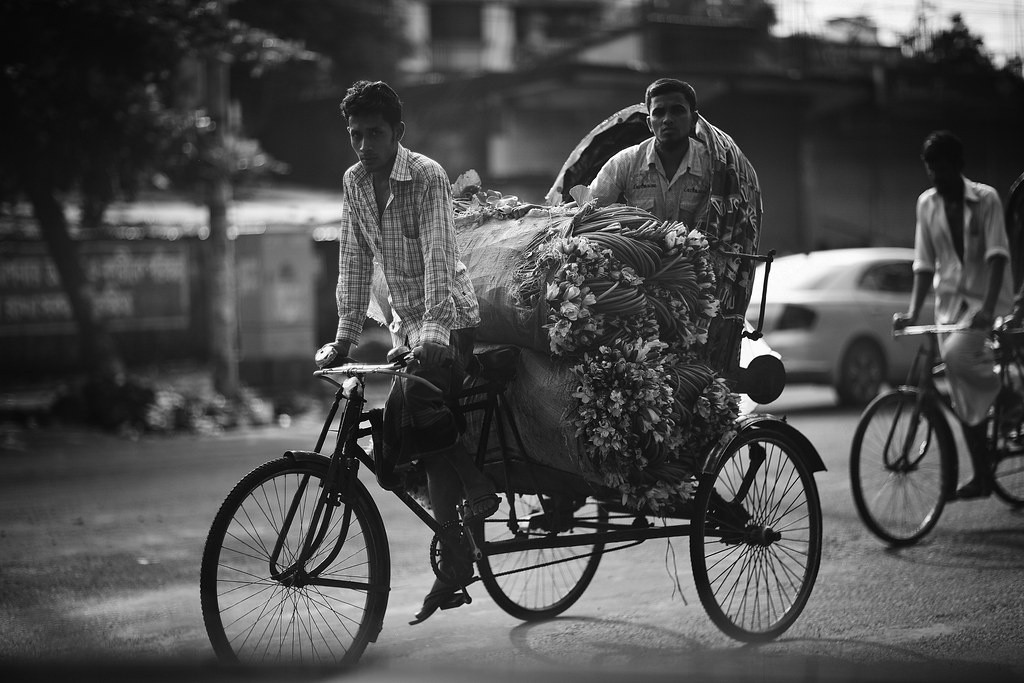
<box><xmin>408</xmin><ymin>575</ymin><xmax>479</xmax><ymax>625</ymax></box>
<box><xmin>461</xmin><ymin>492</ymin><xmax>502</xmax><ymax>526</ymax></box>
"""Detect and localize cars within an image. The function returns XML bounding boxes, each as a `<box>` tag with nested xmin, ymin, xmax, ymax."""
<box><xmin>742</xmin><ymin>245</ymin><xmax>945</xmax><ymax>406</ymax></box>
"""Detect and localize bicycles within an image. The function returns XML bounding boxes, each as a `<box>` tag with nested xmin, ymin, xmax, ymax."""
<box><xmin>846</xmin><ymin>309</ymin><xmax>1024</xmax><ymax>548</ymax></box>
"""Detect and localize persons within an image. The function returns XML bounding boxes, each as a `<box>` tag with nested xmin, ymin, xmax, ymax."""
<box><xmin>315</xmin><ymin>81</ymin><xmax>502</xmax><ymax>631</ymax></box>
<box><xmin>561</xmin><ymin>76</ymin><xmax>714</xmax><ymax>242</ymax></box>
<box><xmin>891</xmin><ymin>129</ymin><xmax>1017</xmax><ymax>502</ymax></box>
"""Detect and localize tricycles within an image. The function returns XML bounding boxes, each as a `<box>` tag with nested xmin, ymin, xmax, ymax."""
<box><xmin>198</xmin><ymin>104</ymin><xmax>829</xmax><ymax>681</ymax></box>
